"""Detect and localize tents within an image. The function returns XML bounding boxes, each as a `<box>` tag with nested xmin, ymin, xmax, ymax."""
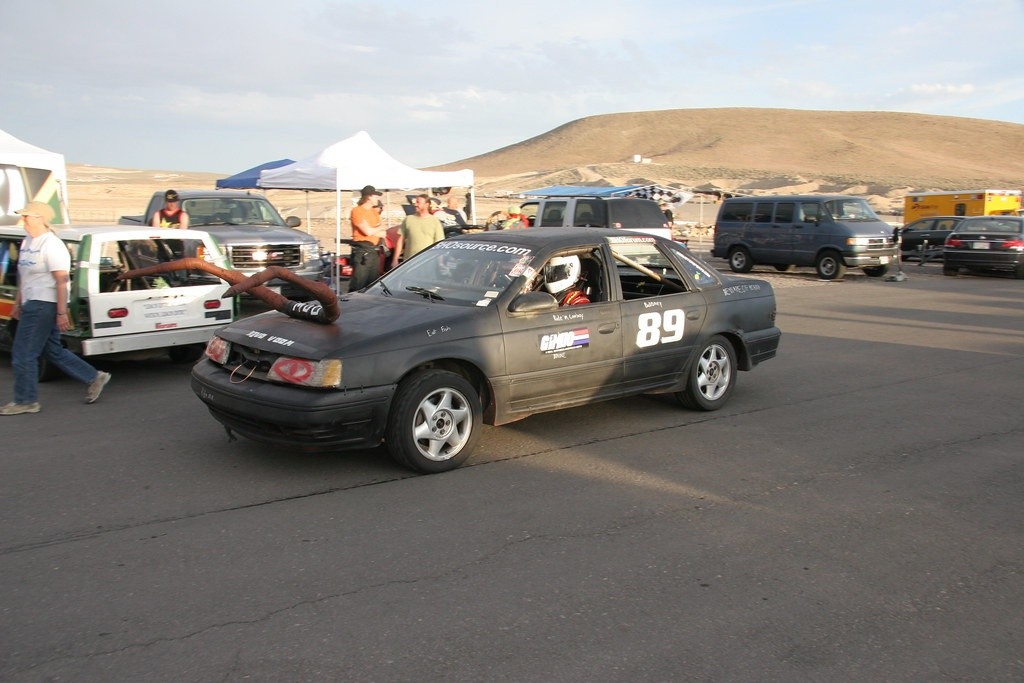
<box><xmin>213</xmin><ymin>130</ymin><xmax>477</xmax><ymax>295</ymax></box>
<box><xmin>511</xmin><ymin>185</ymin><xmax>682</xmax><ymax>197</ymax></box>
<box><xmin>0</xmin><ymin>128</ymin><xmax>70</xmax><ymax>227</ymax></box>
<box><xmin>681</xmin><ymin>183</ymin><xmax>755</xmax><ymax>198</ymax></box>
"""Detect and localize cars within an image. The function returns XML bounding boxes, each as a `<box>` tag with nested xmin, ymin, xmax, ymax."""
<box><xmin>897</xmin><ymin>215</ymin><xmax>999</xmax><ymax>261</ymax></box>
<box><xmin>190</xmin><ymin>221</ymin><xmax>785</xmax><ymax>475</ymax></box>
<box><xmin>941</xmin><ymin>216</ymin><xmax>1024</xmax><ymax>279</ymax></box>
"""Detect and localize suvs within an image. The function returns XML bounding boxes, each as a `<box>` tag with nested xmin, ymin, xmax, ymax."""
<box><xmin>710</xmin><ymin>192</ymin><xmax>900</xmax><ymax>280</ymax></box>
<box><xmin>112</xmin><ymin>188</ymin><xmax>325</xmax><ymax>301</ymax></box>
<box><xmin>516</xmin><ymin>198</ymin><xmax>674</xmax><ymax>264</ymax></box>
<box><xmin>0</xmin><ymin>222</ymin><xmax>241</xmax><ymax>382</ymax></box>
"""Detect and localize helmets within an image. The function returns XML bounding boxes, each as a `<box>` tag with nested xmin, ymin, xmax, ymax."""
<box><xmin>544</xmin><ymin>254</ymin><xmax>582</xmax><ymax>294</ymax></box>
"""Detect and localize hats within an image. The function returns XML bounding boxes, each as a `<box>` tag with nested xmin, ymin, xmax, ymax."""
<box><xmin>362</xmin><ymin>185</ymin><xmax>382</xmax><ymax>196</ymax></box>
<box><xmin>14</xmin><ymin>202</ymin><xmax>55</xmax><ymax>221</ymax></box>
<box><xmin>166</xmin><ymin>190</ymin><xmax>179</xmax><ymax>202</ymax></box>
<box><xmin>508</xmin><ymin>205</ymin><xmax>521</xmax><ymax>215</ymax></box>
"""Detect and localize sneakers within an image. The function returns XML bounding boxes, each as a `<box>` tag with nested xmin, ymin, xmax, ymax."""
<box><xmin>85</xmin><ymin>371</ymin><xmax>111</xmax><ymax>404</ymax></box>
<box><xmin>0</xmin><ymin>401</ymin><xmax>41</xmax><ymax>414</ymax></box>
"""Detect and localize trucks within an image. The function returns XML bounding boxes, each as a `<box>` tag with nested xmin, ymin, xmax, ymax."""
<box><xmin>903</xmin><ymin>187</ymin><xmax>1024</xmax><ymax>230</ymax></box>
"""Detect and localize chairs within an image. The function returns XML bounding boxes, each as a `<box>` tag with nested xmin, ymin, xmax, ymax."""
<box><xmin>575</xmin><ymin>258</ymin><xmax>602</xmax><ymax>303</ymax></box>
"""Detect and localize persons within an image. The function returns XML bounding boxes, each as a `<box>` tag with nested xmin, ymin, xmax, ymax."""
<box><xmin>500</xmin><ymin>206</ymin><xmax>529</xmax><ymax>230</ymax></box>
<box><xmin>373</xmin><ymin>200</ymin><xmax>384</xmax><ymax>215</ymax></box>
<box><xmin>347</xmin><ymin>186</ymin><xmax>390</xmax><ymax>294</ymax></box>
<box><xmin>0</xmin><ymin>201</ymin><xmax>112</xmax><ymax>416</ymax></box>
<box><xmin>391</xmin><ymin>194</ymin><xmax>449</xmax><ymax>277</ymax></box>
<box><xmin>151</xmin><ymin>190</ymin><xmax>189</xmax><ymax>230</ymax></box>
<box><xmin>534</xmin><ymin>254</ymin><xmax>591</xmax><ymax>307</ymax></box>
